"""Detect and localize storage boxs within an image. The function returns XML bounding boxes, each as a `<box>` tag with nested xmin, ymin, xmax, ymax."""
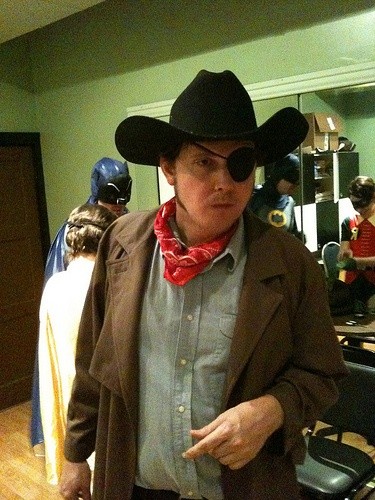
<box><xmin>303</xmin><ymin>112</ymin><xmax>343</xmax><ymax>151</ymax></box>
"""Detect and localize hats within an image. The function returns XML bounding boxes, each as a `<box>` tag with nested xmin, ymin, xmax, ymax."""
<box><xmin>115</xmin><ymin>69</ymin><xmax>309</xmax><ymax>167</ymax></box>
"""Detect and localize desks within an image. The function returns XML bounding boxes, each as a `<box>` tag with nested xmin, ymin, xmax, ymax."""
<box><xmin>332</xmin><ymin>313</ymin><xmax>375</xmax><ymax>347</ymax></box>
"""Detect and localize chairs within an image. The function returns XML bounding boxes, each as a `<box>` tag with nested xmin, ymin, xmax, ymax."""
<box><xmin>295</xmin><ymin>361</ymin><xmax>375</xmax><ymax>500</ymax></box>
<box><xmin>321</xmin><ymin>242</ymin><xmax>340</xmax><ymax>282</ymax></box>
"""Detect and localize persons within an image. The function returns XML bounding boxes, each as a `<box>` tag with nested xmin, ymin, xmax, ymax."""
<box><xmin>29</xmin><ymin>155</ymin><xmax>133</xmax><ymax>486</ymax></box>
<box><xmin>249</xmin><ymin>153</ymin><xmax>374</xmax><ymax>285</ymax></box>
<box><xmin>59</xmin><ymin>70</ymin><xmax>348</xmax><ymax>499</ymax></box>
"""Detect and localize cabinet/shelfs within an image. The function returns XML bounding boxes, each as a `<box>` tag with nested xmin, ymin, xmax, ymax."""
<box><xmin>264</xmin><ymin>151</ymin><xmax>360</xmax><ymax>206</ymax></box>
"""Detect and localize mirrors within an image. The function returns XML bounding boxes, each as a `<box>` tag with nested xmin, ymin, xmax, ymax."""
<box><xmin>126</xmin><ymin>59</ymin><xmax>375</xmax><ymax>366</ymax></box>
